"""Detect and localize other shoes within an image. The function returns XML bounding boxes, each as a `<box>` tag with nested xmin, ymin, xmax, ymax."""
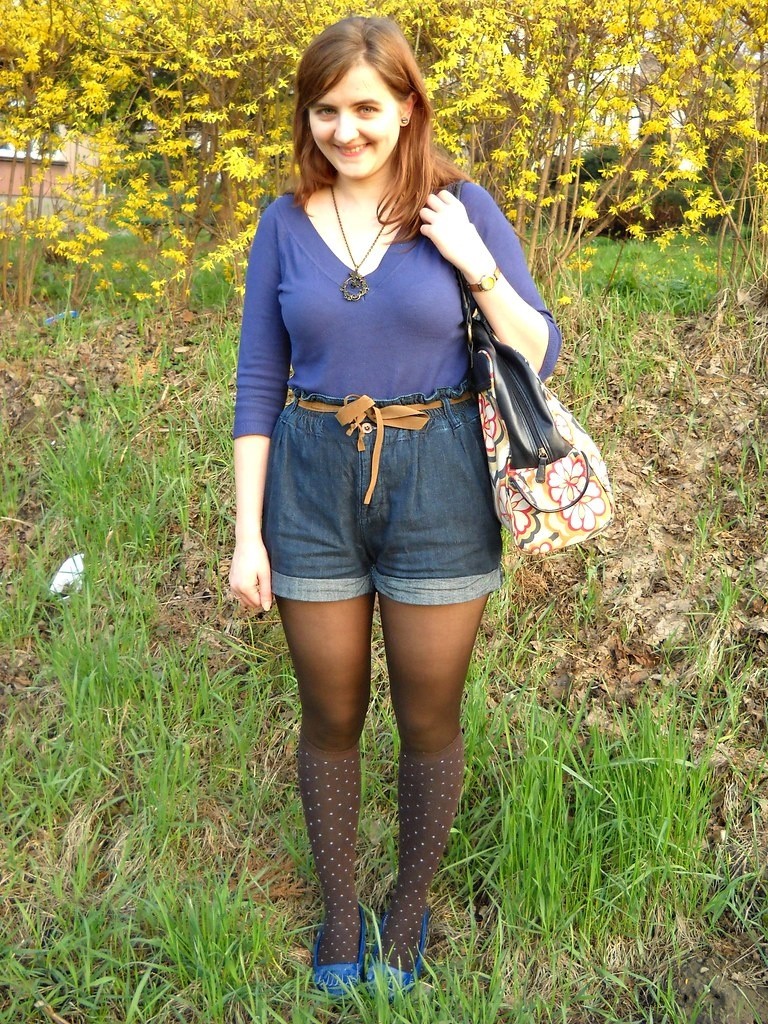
<box><xmin>366</xmin><ymin>909</ymin><xmax>430</xmax><ymax>1002</ymax></box>
<box><xmin>314</xmin><ymin>903</ymin><xmax>364</xmax><ymax>996</ymax></box>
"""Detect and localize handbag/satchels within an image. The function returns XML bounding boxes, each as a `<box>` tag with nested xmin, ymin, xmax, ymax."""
<box><xmin>442</xmin><ymin>179</ymin><xmax>614</xmax><ymax>558</ymax></box>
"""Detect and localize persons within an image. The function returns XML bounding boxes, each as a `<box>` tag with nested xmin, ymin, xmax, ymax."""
<box><xmin>228</xmin><ymin>14</ymin><xmax>560</xmax><ymax>1001</ymax></box>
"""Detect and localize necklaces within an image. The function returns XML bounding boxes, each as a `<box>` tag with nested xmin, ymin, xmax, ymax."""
<box><xmin>330</xmin><ymin>183</ymin><xmax>399</xmax><ymax>300</ymax></box>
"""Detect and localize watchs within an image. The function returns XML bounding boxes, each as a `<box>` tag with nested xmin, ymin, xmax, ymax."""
<box><xmin>469</xmin><ymin>267</ymin><xmax>500</xmax><ymax>291</ymax></box>
